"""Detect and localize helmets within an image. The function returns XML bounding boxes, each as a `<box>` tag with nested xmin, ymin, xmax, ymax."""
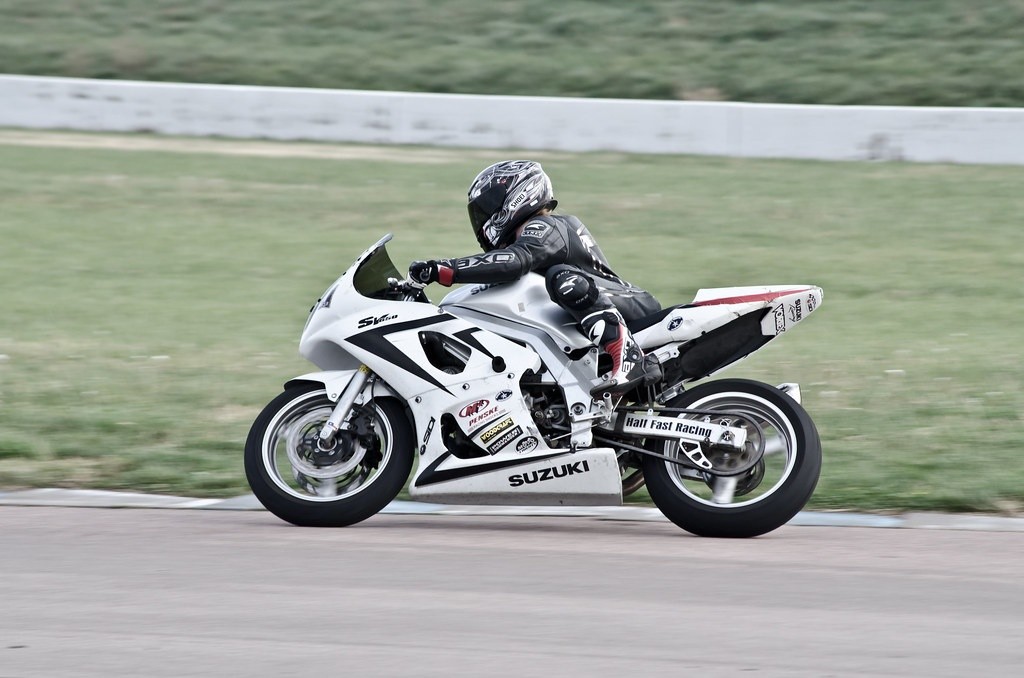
<box><xmin>467</xmin><ymin>159</ymin><xmax>558</xmax><ymax>253</ymax></box>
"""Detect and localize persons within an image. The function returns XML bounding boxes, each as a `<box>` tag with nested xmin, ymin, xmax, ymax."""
<box><xmin>407</xmin><ymin>158</ymin><xmax>663</xmax><ymax>400</ymax></box>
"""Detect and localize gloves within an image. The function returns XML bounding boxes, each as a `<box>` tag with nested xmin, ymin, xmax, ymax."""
<box><xmin>408</xmin><ymin>257</ymin><xmax>459</xmax><ymax>287</ymax></box>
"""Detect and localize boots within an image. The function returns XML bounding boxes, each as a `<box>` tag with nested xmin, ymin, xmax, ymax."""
<box><xmin>580</xmin><ymin>307</ymin><xmax>663</xmax><ymax>400</ymax></box>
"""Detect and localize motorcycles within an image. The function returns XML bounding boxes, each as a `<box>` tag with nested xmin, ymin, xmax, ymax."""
<box><xmin>244</xmin><ymin>233</ymin><xmax>827</xmax><ymax>539</ymax></box>
